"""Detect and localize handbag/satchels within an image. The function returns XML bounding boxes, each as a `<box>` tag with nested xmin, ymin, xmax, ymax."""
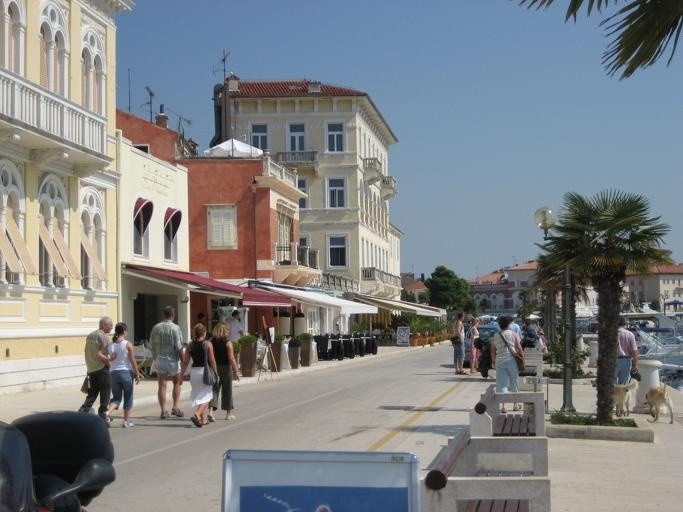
<box><xmin>450</xmin><ymin>336</ymin><xmax>460</xmax><ymax>344</ymax></box>
<box><xmin>81</xmin><ymin>378</ymin><xmax>91</xmax><ymax>393</ymax></box>
<box><xmin>204</xmin><ymin>362</ymin><xmax>218</xmax><ymax>385</ymax></box>
<box><xmin>514</xmin><ymin>352</ymin><xmax>525</xmax><ymax>371</ymax></box>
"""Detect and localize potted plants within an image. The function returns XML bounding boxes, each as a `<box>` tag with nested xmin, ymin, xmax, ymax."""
<box><xmin>409</xmin><ymin>314</ymin><xmax>453</xmax><ymax>347</ymax></box>
<box><xmin>232</xmin><ymin>333</ymin><xmax>317</xmax><ymax>380</ymax></box>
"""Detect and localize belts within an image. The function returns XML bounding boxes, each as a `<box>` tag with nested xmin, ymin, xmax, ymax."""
<box><xmin>619</xmin><ymin>356</ymin><xmax>631</xmax><ymax>358</ymax></box>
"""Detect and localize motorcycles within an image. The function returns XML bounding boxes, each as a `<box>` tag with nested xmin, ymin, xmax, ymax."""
<box><xmin>0</xmin><ymin>409</ymin><xmax>114</xmax><ymax>512</ymax></box>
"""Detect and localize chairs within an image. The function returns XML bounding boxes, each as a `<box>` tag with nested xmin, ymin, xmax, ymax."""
<box><xmin>421</xmin><ymin>430</ymin><xmax>551</xmax><ymax>512</ymax></box>
<box><xmin>469</xmin><ymin>391</ymin><xmax>544</xmax><ymax>436</ymax></box>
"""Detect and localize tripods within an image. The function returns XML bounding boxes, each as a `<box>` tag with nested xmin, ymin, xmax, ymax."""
<box><xmin>258</xmin><ymin>345</ymin><xmax>281</xmax><ymax>382</ymax></box>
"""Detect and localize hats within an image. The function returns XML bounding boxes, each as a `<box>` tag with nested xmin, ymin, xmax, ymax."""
<box><xmin>194</xmin><ymin>323</ymin><xmax>207</xmax><ymax>338</ymax></box>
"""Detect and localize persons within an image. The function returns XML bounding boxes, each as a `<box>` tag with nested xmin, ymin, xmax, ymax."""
<box><xmin>612</xmin><ymin>315</ymin><xmax>638</xmax><ymax>385</ymax></box>
<box><xmin>226</xmin><ymin>310</ymin><xmax>243</xmax><ymax>341</ymax></box>
<box><xmin>79</xmin><ymin>317</ymin><xmax>113</xmax><ymax>421</ymax></box>
<box><xmin>97</xmin><ymin>323</ymin><xmax>140</xmax><ymax>427</ymax></box>
<box><xmin>207</xmin><ymin>322</ymin><xmax>240</xmax><ymax>422</ymax></box>
<box><xmin>193</xmin><ymin>313</ymin><xmax>207</xmax><ymax>325</ymax></box>
<box><xmin>491</xmin><ymin>316</ymin><xmax>523</xmax><ymax>413</ymax></box>
<box><xmin>452</xmin><ymin>311</ymin><xmax>544</xmax><ymax>376</ymax></box>
<box><xmin>152</xmin><ymin>305</ymin><xmax>184</xmax><ymax>419</ymax></box>
<box><xmin>180</xmin><ymin>324</ymin><xmax>219</xmax><ymax>427</ymax></box>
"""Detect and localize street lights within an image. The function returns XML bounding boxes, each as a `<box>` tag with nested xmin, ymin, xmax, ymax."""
<box><xmin>532</xmin><ymin>206</ymin><xmax>577</xmax><ymax>414</ymax></box>
<box><xmin>534</xmin><ymin>275</ymin><xmax>557</xmax><ymax>347</ymax></box>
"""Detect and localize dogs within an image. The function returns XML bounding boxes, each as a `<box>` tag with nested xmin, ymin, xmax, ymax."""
<box><xmin>644</xmin><ymin>383</ymin><xmax>675</xmax><ymax>425</ymax></box>
<box><xmin>589</xmin><ymin>376</ymin><xmax>639</xmax><ymax>417</ymax></box>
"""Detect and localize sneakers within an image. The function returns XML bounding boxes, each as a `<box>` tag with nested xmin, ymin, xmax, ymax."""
<box><xmin>123</xmin><ymin>421</ymin><xmax>135</xmax><ymax>428</ymax></box>
<box><xmin>161</xmin><ymin>408</ymin><xmax>236</xmax><ymax>427</ymax></box>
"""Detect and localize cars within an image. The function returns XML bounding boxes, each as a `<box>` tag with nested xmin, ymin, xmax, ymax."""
<box><xmin>461</xmin><ymin>318</ymin><xmax>522</xmax><ymax>334</ymax></box>
<box><xmin>463</xmin><ymin>324</ymin><xmax>500</xmax><ymax>365</ymax></box>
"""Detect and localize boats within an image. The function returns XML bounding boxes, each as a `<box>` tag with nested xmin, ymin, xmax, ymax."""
<box><xmin>575</xmin><ymin>300</ymin><xmax>683</xmax><ymax>383</ymax></box>
